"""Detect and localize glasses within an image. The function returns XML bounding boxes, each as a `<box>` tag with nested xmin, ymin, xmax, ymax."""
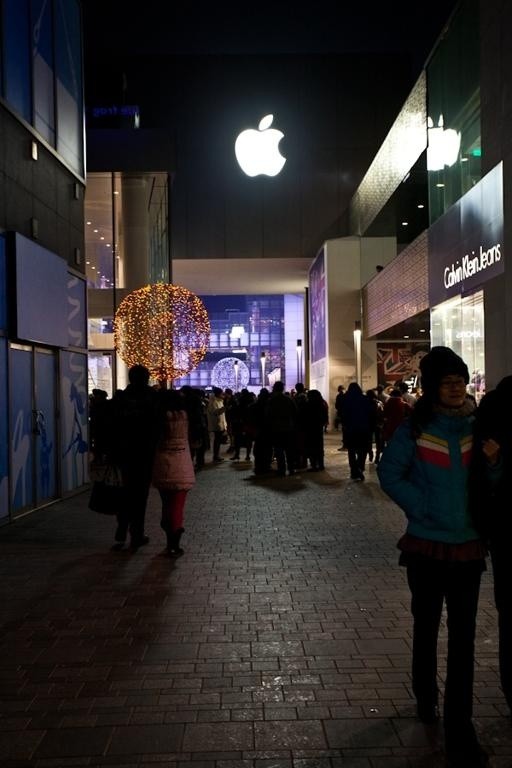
<box><xmin>440</xmin><ymin>377</ymin><xmax>466</xmax><ymax>389</ymax></box>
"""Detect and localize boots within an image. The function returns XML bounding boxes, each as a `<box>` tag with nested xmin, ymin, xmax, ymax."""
<box><xmin>161</xmin><ymin>528</ymin><xmax>186</xmax><ymax>560</ymax></box>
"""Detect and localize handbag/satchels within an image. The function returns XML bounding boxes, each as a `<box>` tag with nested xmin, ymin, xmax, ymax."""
<box><xmin>88</xmin><ymin>480</ymin><xmax>122</xmax><ymax>515</ymax></box>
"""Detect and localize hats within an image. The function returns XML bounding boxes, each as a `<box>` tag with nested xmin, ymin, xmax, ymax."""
<box><xmin>419</xmin><ymin>347</ymin><xmax>469</xmax><ymax>394</ymax></box>
